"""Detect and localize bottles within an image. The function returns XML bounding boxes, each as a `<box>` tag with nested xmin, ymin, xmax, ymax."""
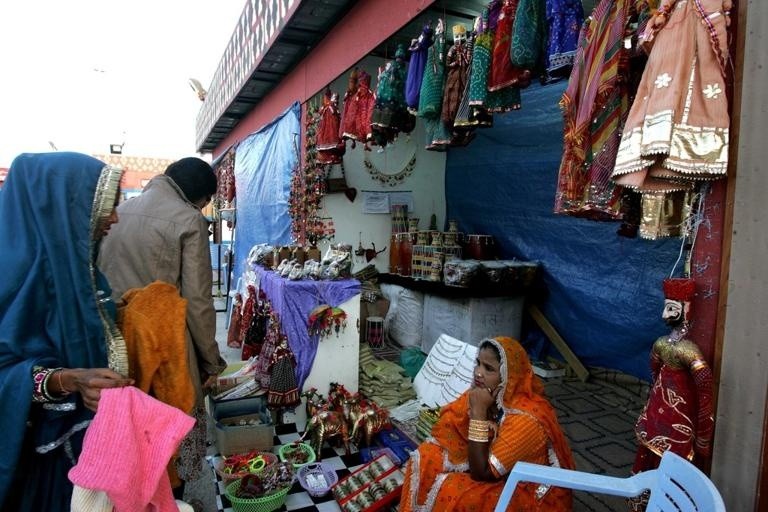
<box><xmin>278</xmin><ymin>245</ymin><xmax>320</xmax><ymax>264</ymax></box>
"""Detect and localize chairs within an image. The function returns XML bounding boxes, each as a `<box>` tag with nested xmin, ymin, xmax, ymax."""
<box><xmin>493</xmin><ymin>451</ymin><xmax>725</xmax><ymax>511</ymax></box>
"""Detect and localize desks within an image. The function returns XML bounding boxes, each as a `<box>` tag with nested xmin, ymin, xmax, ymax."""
<box><xmin>244</xmin><ymin>260</ymin><xmax>362</xmax><ymax>433</ymax></box>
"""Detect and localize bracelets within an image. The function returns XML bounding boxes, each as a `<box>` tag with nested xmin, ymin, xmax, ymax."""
<box><xmin>467</xmin><ymin>419</ymin><xmax>489</xmax><ymax>432</ymax></box>
<box><xmin>467</xmin><ymin>430</ymin><xmax>489</xmax><ymax>442</ymax></box>
<box><xmin>58</xmin><ymin>369</ymin><xmax>73</xmax><ymax>395</ymax></box>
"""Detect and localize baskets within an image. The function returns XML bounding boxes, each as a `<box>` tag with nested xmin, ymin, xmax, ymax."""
<box><xmin>279</xmin><ymin>442</ymin><xmax>317</xmax><ymax>473</ymax></box>
<box><xmin>297</xmin><ymin>462</ymin><xmax>338</xmax><ymax>498</ymax></box>
<box><xmin>224</xmin><ymin>473</ymin><xmax>292</xmax><ymax>512</ymax></box>
<box><xmin>214</xmin><ymin>452</ymin><xmax>278</xmax><ymax>484</ymax></box>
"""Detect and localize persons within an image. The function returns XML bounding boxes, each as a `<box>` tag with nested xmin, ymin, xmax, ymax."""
<box><xmin>624</xmin><ymin>277</ymin><xmax>716</xmax><ymax>512</ymax></box>
<box><xmin>0</xmin><ymin>151</ymin><xmax>136</xmax><ymax>512</ymax></box>
<box><xmin>95</xmin><ymin>157</ymin><xmax>226</xmax><ymax>512</ymax></box>
<box><xmin>400</xmin><ymin>336</ymin><xmax>577</xmax><ymax>512</ymax></box>
